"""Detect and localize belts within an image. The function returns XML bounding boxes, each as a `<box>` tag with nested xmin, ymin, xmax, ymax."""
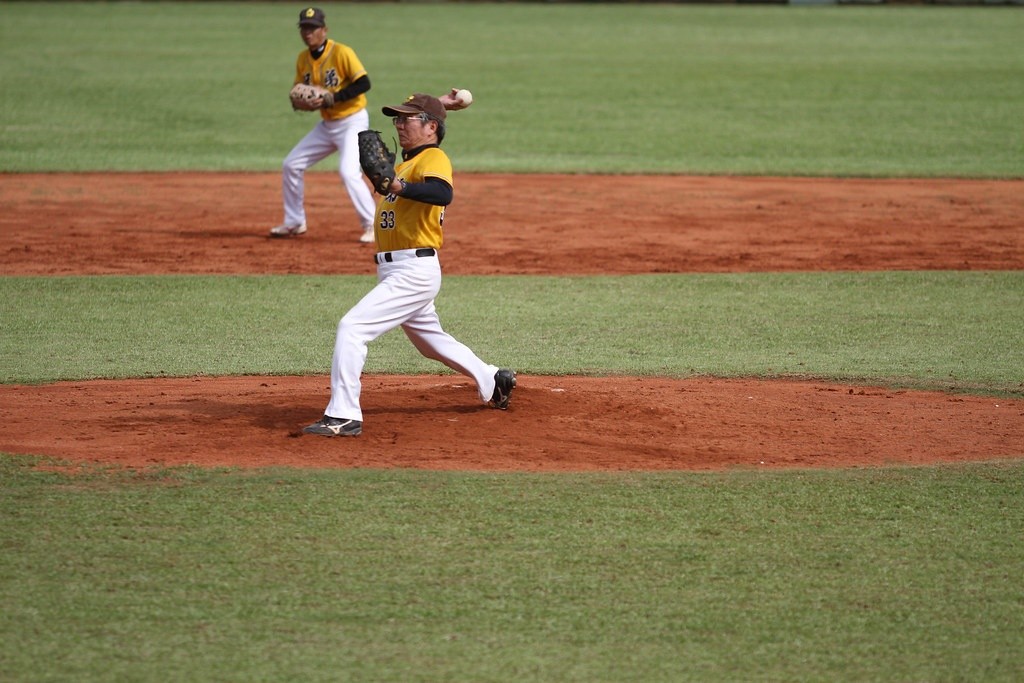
<box><xmin>374</xmin><ymin>249</ymin><xmax>435</xmax><ymax>264</ymax></box>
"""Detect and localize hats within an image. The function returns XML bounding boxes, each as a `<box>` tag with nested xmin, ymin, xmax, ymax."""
<box><xmin>382</xmin><ymin>93</ymin><xmax>446</xmax><ymax>121</ymax></box>
<box><xmin>299</xmin><ymin>7</ymin><xmax>325</xmax><ymax>27</ymax></box>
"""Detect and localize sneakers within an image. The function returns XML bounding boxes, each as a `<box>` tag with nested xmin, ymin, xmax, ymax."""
<box><xmin>359</xmin><ymin>229</ymin><xmax>375</xmax><ymax>242</ymax></box>
<box><xmin>492</xmin><ymin>369</ymin><xmax>517</xmax><ymax>410</ymax></box>
<box><xmin>270</xmin><ymin>224</ymin><xmax>307</xmax><ymax>236</ymax></box>
<box><xmin>303</xmin><ymin>415</ymin><xmax>362</xmax><ymax>438</ymax></box>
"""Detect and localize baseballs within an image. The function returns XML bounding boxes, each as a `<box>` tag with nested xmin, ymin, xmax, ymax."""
<box><xmin>455</xmin><ymin>89</ymin><xmax>472</xmax><ymax>107</ymax></box>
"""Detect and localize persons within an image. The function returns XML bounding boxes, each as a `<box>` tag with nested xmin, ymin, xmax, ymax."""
<box><xmin>269</xmin><ymin>6</ymin><xmax>376</xmax><ymax>241</ymax></box>
<box><xmin>302</xmin><ymin>87</ymin><xmax>517</xmax><ymax>436</ymax></box>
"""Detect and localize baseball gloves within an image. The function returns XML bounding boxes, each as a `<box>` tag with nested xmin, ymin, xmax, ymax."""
<box><xmin>289</xmin><ymin>82</ymin><xmax>334</xmax><ymax>113</ymax></box>
<box><xmin>356</xmin><ymin>129</ymin><xmax>399</xmax><ymax>196</ymax></box>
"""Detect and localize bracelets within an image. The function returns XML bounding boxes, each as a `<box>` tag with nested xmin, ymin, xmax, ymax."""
<box><xmin>398</xmin><ymin>178</ymin><xmax>406</xmax><ymax>194</ymax></box>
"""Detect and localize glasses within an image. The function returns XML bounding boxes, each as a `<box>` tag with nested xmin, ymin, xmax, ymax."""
<box><xmin>393</xmin><ymin>117</ymin><xmax>421</xmax><ymax>125</ymax></box>
